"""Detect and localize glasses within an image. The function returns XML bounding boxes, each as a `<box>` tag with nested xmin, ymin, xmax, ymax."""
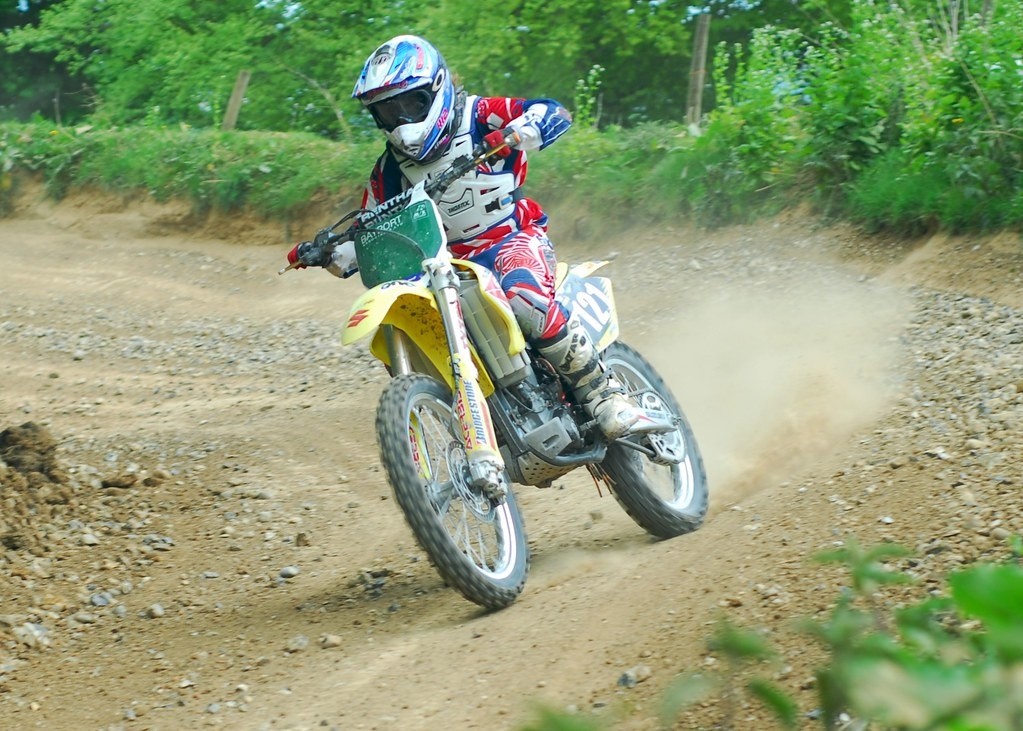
<box><xmin>369</xmin><ymin>89</ymin><xmax>432</xmax><ymax>129</ymax></box>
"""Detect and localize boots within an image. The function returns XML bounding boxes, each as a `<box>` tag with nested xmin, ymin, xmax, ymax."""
<box><xmin>536</xmin><ymin>306</ymin><xmax>637</xmax><ymax>440</ymax></box>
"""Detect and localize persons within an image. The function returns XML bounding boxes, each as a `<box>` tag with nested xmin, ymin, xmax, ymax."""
<box><xmin>287</xmin><ymin>34</ymin><xmax>639</xmax><ymax>440</ymax></box>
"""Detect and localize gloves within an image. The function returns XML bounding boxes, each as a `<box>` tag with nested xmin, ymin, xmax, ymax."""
<box><xmin>288</xmin><ymin>241</ymin><xmax>322</xmax><ymax>269</ymax></box>
<box><xmin>470</xmin><ymin>124</ymin><xmax>518</xmax><ymax>165</ymax></box>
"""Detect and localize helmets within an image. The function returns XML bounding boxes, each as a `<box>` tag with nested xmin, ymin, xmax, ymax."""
<box><xmin>351</xmin><ymin>33</ymin><xmax>460</xmax><ymax>167</ymax></box>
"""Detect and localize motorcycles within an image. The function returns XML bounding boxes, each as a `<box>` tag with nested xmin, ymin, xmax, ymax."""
<box><xmin>277</xmin><ymin>133</ymin><xmax>709</xmax><ymax>610</ymax></box>
